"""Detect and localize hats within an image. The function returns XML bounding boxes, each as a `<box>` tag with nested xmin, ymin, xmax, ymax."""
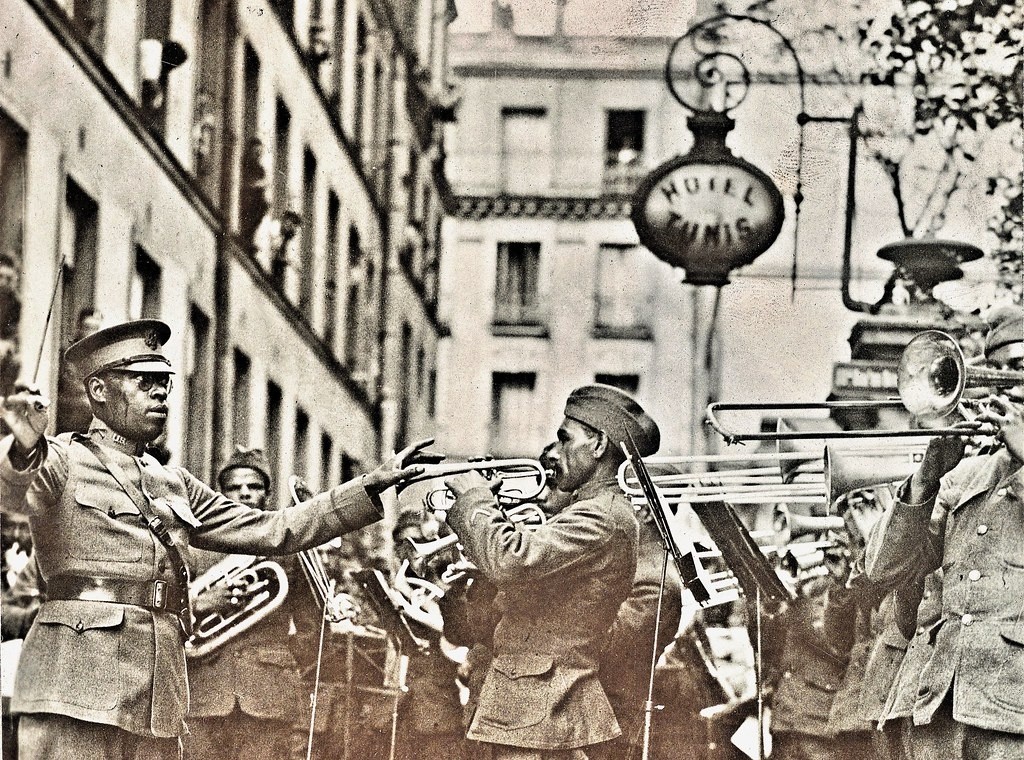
<box><xmin>218</xmin><ymin>444</ymin><xmax>271</xmax><ymax>491</ymax></box>
<box><xmin>64</xmin><ymin>319</ymin><xmax>175</xmax><ymax>382</ymax></box>
<box><xmin>564</xmin><ymin>384</ymin><xmax>660</xmax><ymax>459</ymax></box>
<box><xmin>984</xmin><ymin>305</ymin><xmax>1024</xmax><ymax>359</ymax></box>
<box><xmin>623</xmin><ymin>462</ymin><xmax>688</xmax><ymax>502</ymax></box>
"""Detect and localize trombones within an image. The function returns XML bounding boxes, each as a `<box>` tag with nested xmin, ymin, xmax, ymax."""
<box><xmin>619</xmin><ymin>329</ymin><xmax>1024</xmax><ymax>602</ymax></box>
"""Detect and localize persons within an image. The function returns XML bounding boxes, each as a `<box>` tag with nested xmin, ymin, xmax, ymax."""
<box><xmin>3</xmin><ymin>443</ymin><xmax>717</xmax><ymax>760</ymax></box>
<box><xmin>763</xmin><ymin>317</ymin><xmax>1023</xmax><ymax>758</ymax></box>
<box><xmin>1</xmin><ymin>313</ymin><xmax>436</xmax><ymax>760</ymax></box>
<box><xmin>436</xmin><ymin>382</ymin><xmax>663</xmax><ymax>760</ymax></box>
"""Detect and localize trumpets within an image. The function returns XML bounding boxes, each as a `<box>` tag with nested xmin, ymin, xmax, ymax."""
<box><xmin>393</xmin><ymin>455</ymin><xmax>557</xmax><ymax>510</ymax></box>
<box><xmin>401</xmin><ymin>496</ymin><xmax>552</xmax><ymax>582</ymax></box>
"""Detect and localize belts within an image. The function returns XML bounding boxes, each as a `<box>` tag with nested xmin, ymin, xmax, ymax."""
<box><xmin>46</xmin><ymin>577</ymin><xmax>181</xmax><ymax>613</ymax></box>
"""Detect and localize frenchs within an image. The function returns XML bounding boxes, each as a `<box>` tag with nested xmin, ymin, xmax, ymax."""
<box><xmin>184</xmin><ymin>479</ymin><xmax>323</xmax><ymax>664</ymax></box>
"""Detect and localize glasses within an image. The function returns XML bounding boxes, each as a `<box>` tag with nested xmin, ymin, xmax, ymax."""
<box><xmin>138</xmin><ymin>375</ymin><xmax>169</xmax><ymax>391</ymax></box>
